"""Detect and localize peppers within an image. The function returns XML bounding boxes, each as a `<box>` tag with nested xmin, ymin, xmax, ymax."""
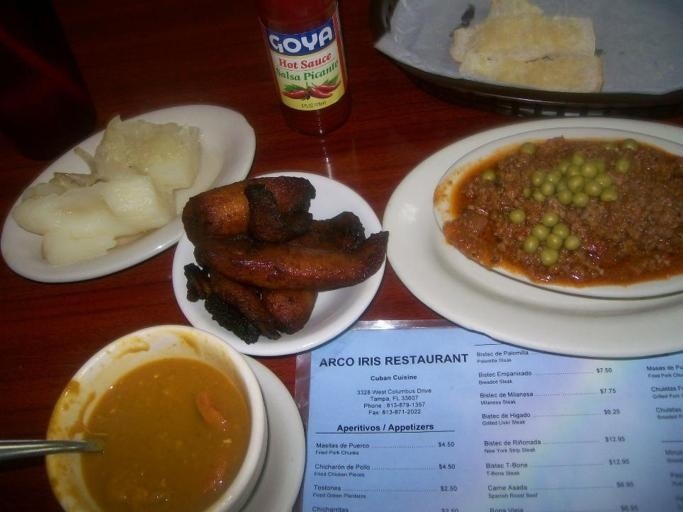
<box><xmin>282</xmin><ymin>82</ymin><xmax>339</xmax><ymax>99</ymax></box>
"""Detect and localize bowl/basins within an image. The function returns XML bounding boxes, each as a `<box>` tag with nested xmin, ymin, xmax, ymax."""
<box><xmin>44</xmin><ymin>324</ymin><xmax>270</xmax><ymax>512</ymax></box>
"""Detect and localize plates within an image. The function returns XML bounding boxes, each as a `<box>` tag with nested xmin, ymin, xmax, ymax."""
<box><xmin>241</xmin><ymin>353</ymin><xmax>306</xmax><ymax>512</ymax></box>
<box><xmin>170</xmin><ymin>169</ymin><xmax>387</xmax><ymax>358</ymax></box>
<box><xmin>383</xmin><ymin>116</ymin><xmax>683</xmax><ymax>359</ymax></box>
<box><xmin>0</xmin><ymin>102</ymin><xmax>259</xmax><ymax>284</ymax></box>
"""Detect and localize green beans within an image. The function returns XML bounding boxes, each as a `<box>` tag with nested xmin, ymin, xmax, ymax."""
<box><xmin>480</xmin><ymin>139</ymin><xmax>639</xmax><ymax>264</ymax></box>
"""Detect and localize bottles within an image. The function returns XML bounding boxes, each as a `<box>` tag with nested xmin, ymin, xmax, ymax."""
<box><xmin>257</xmin><ymin>0</ymin><xmax>353</xmax><ymax>136</ymax></box>
